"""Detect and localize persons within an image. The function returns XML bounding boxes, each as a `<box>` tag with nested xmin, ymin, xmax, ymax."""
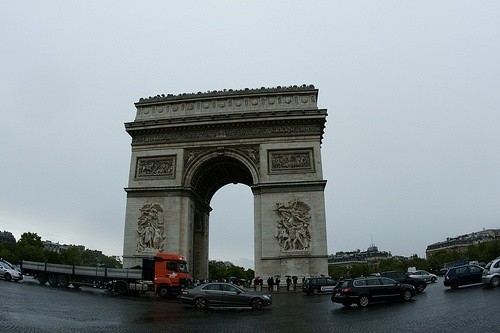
<box><xmin>275</xmin><ymin>278</ymin><xmax>280</xmax><ymax>289</ymax></box>
<box><xmin>303</xmin><ymin>277</ymin><xmax>305</xmax><ymax>283</ymax></box>
<box><xmin>267</xmin><ymin>277</ymin><xmax>274</xmax><ymax>292</ymax></box>
<box><xmin>188</xmin><ymin>278</ymin><xmax>238</xmax><ymax>286</ymax></box>
<box><xmin>254</xmin><ymin>279</ymin><xmax>263</xmax><ymax>290</ymax></box>
<box><xmin>293</xmin><ymin>277</ymin><xmax>297</xmax><ymax>289</ymax></box>
<box><xmin>286</xmin><ymin>277</ymin><xmax>292</xmax><ymax>290</ymax></box>
<box><xmin>239</xmin><ymin>280</ymin><xmax>250</xmax><ymax>288</ymax></box>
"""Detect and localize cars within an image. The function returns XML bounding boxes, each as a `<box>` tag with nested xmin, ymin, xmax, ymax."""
<box><xmin>444</xmin><ymin>265</ymin><xmax>484</xmax><ymax>289</ymax></box>
<box><xmin>0</xmin><ymin>262</ymin><xmax>25</xmax><ymax>282</ymax></box>
<box><xmin>482</xmin><ymin>259</ymin><xmax>500</xmax><ymax>287</ymax></box>
<box><xmin>186</xmin><ymin>284</ymin><xmax>273</xmax><ymax>311</ymax></box>
<box><xmin>334</xmin><ymin>271</ymin><xmax>439</xmax><ymax>309</ymax></box>
<box><xmin>304</xmin><ymin>278</ymin><xmax>339</xmax><ymax>294</ymax></box>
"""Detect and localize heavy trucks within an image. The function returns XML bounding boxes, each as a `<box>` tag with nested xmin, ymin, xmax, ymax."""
<box><xmin>23</xmin><ymin>254</ymin><xmax>191</xmax><ymax>299</ymax></box>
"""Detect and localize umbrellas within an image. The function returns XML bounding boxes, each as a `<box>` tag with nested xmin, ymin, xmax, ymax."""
<box><xmin>285</xmin><ymin>275</ymin><xmax>291</xmax><ymax>278</ymax></box>
<box><xmin>275</xmin><ymin>275</ymin><xmax>281</xmax><ymax>277</ymax></box>
<box><xmin>229</xmin><ymin>277</ymin><xmax>237</xmax><ymax>278</ymax></box>
<box><xmin>292</xmin><ymin>276</ymin><xmax>298</xmax><ymax>279</ymax></box>
<box><xmin>240</xmin><ymin>279</ymin><xmax>247</xmax><ymax>281</ymax></box>
<box><xmin>254</xmin><ymin>276</ymin><xmax>260</xmax><ymax>280</ymax></box>
